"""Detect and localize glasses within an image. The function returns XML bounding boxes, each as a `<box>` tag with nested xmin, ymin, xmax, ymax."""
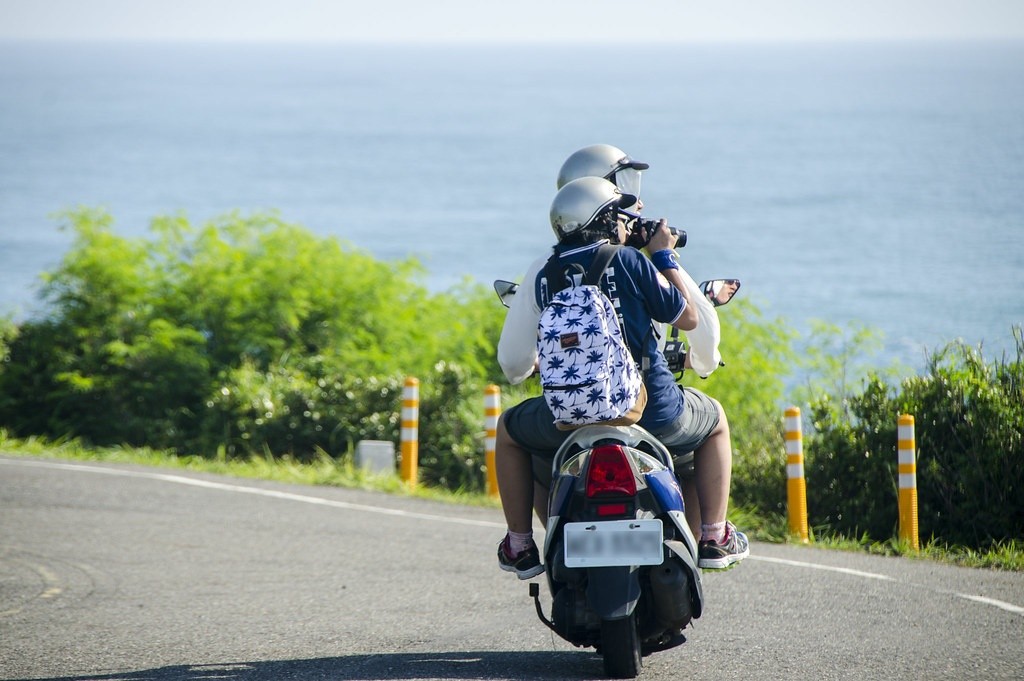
<box><xmin>617</xmin><ymin>216</ymin><xmax>630</xmax><ymax>232</ymax></box>
<box><xmin>725</xmin><ymin>280</ymin><xmax>740</xmax><ymax>287</ymax></box>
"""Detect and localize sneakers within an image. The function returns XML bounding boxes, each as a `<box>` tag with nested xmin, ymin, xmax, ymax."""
<box><xmin>698</xmin><ymin>520</ymin><xmax>750</xmax><ymax>568</ymax></box>
<box><xmin>498</xmin><ymin>532</ymin><xmax>544</xmax><ymax>580</ymax></box>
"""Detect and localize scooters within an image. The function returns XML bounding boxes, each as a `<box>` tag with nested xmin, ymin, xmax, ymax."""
<box><xmin>494</xmin><ymin>279</ymin><xmax>742</xmax><ymax>678</ymax></box>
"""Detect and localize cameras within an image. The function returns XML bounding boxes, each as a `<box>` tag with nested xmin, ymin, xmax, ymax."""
<box><xmin>628</xmin><ymin>220</ymin><xmax>687</xmax><ymax>252</ymax></box>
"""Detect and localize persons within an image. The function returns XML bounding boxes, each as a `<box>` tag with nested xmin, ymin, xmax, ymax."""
<box><xmin>707</xmin><ymin>279</ymin><xmax>739</xmax><ymax>307</ymax></box>
<box><xmin>496</xmin><ymin>145</ymin><xmax>749</xmax><ymax>581</ymax></box>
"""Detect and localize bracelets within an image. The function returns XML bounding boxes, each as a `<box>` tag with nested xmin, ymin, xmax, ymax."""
<box><xmin>652</xmin><ymin>249</ymin><xmax>678</xmax><ymax>273</ymax></box>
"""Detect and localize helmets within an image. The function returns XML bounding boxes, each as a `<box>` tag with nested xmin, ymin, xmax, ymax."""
<box><xmin>558</xmin><ymin>144</ymin><xmax>649</xmax><ymax>190</ymax></box>
<box><xmin>550</xmin><ymin>176</ymin><xmax>637</xmax><ymax>242</ymax></box>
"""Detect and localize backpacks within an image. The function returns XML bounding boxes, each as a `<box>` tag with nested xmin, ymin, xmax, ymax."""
<box><xmin>536</xmin><ymin>244</ymin><xmax>648</xmax><ymax>430</ymax></box>
